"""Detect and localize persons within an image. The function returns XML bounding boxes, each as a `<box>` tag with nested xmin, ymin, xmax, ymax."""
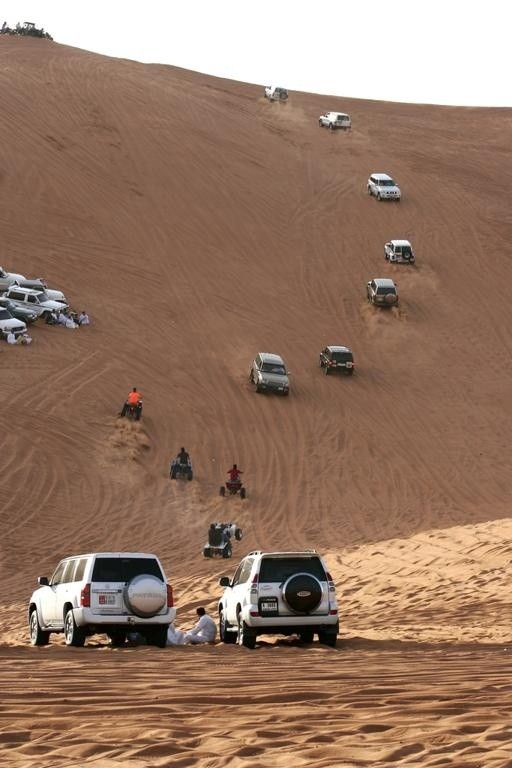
<box><xmin>186</xmin><ymin>608</ymin><xmax>217</xmax><ymax>645</ymax></box>
<box><xmin>227</xmin><ymin>465</ymin><xmax>243</xmax><ymax>482</ymax></box>
<box><xmin>175</xmin><ymin>448</ymin><xmax>189</xmax><ymax>467</ymax></box>
<box><xmin>46</xmin><ymin>308</ymin><xmax>90</xmax><ymax>329</ymax></box>
<box><xmin>6</xmin><ymin>328</ymin><xmax>32</xmax><ymax>345</ymax></box>
<box><xmin>208</xmin><ymin>524</ymin><xmax>225</xmax><ymax>547</ymax></box>
<box><xmin>2</xmin><ymin>22</ymin><xmax>50</xmax><ymax>38</ymax></box>
<box><xmin>127</xmin><ymin>388</ymin><xmax>142</xmax><ymax>407</ymax></box>
<box><xmin>167</xmin><ymin>623</ymin><xmax>192</xmax><ymax>645</ymax></box>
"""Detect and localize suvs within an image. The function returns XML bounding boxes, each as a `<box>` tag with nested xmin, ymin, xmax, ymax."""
<box><xmin>317</xmin><ymin>345</ymin><xmax>358</xmax><ymax>377</ymax></box>
<box><xmin>218</xmin><ymin>547</ymin><xmax>340</xmax><ymax>650</ymax></box>
<box><xmin>248</xmin><ymin>351</ymin><xmax>289</xmax><ymax>397</ymax></box>
<box><xmin>202</xmin><ymin>521</ymin><xmax>243</xmax><ymax>558</ymax></box>
<box><xmin>0</xmin><ymin>267</ymin><xmax>67</xmax><ymax>338</ymax></box>
<box><xmin>366</xmin><ymin>277</ymin><xmax>403</xmax><ymax>310</ymax></box>
<box><xmin>119</xmin><ymin>400</ymin><xmax>143</xmax><ymax>419</ymax></box>
<box><xmin>367</xmin><ymin>173</ymin><xmax>400</xmax><ymax>204</ymax></box>
<box><xmin>170</xmin><ymin>460</ymin><xmax>193</xmax><ymax>481</ymax></box>
<box><xmin>28</xmin><ymin>550</ymin><xmax>175</xmax><ymax>648</ymax></box>
<box><xmin>384</xmin><ymin>239</ymin><xmax>414</xmax><ymax>269</ymax></box>
<box><xmin>262</xmin><ymin>84</ymin><xmax>289</xmax><ymax>104</ymax></box>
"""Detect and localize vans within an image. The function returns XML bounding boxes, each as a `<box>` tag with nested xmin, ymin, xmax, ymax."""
<box><xmin>317</xmin><ymin>111</ymin><xmax>351</xmax><ymax>129</ymax></box>
